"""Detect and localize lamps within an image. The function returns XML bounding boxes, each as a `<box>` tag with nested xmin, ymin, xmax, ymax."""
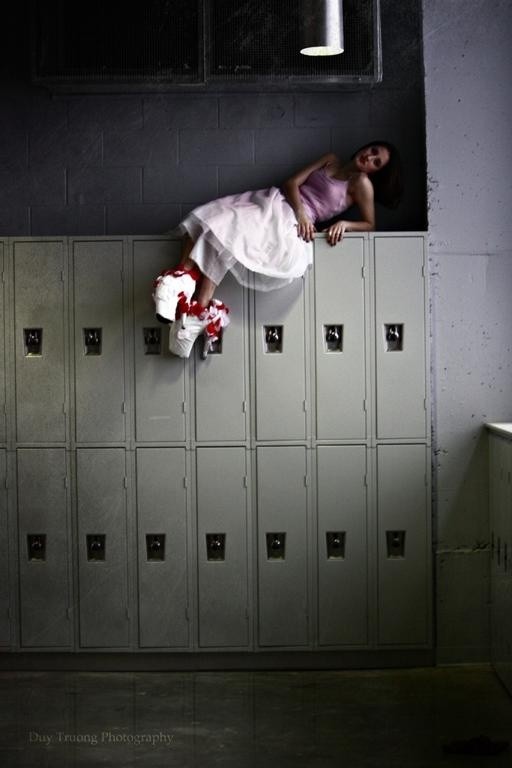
<box><xmin>297</xmin><ymin>1</ymin><xmax>345</xmax><ymax>57</ymax></box>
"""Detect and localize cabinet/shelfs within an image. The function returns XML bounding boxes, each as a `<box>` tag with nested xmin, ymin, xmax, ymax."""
<box><xmin>484</xmin><ymin>422</ymin><xmax>512</xmax><ymax>694</ymax></box>
<box><xmin>1</xmin><ymin>228</ymin><xmax>435</xmax><ymax>665</ymax></box>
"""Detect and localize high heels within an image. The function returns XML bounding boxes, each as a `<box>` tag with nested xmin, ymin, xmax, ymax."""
<box><xmin>168</xmin><ymin>315</ymin><xmax>213</xmax><ymax>360</ymax></box>
<box><xmin>155</xmin><ymin>273</ymin><xmax>196</xmax><ymax>324</ymax></box>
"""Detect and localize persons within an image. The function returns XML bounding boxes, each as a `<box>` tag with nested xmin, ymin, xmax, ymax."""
<box><xmin>151</xmin><ymin>139</ymin><xmax>404</xmax><ymax>360</ymax></box>
